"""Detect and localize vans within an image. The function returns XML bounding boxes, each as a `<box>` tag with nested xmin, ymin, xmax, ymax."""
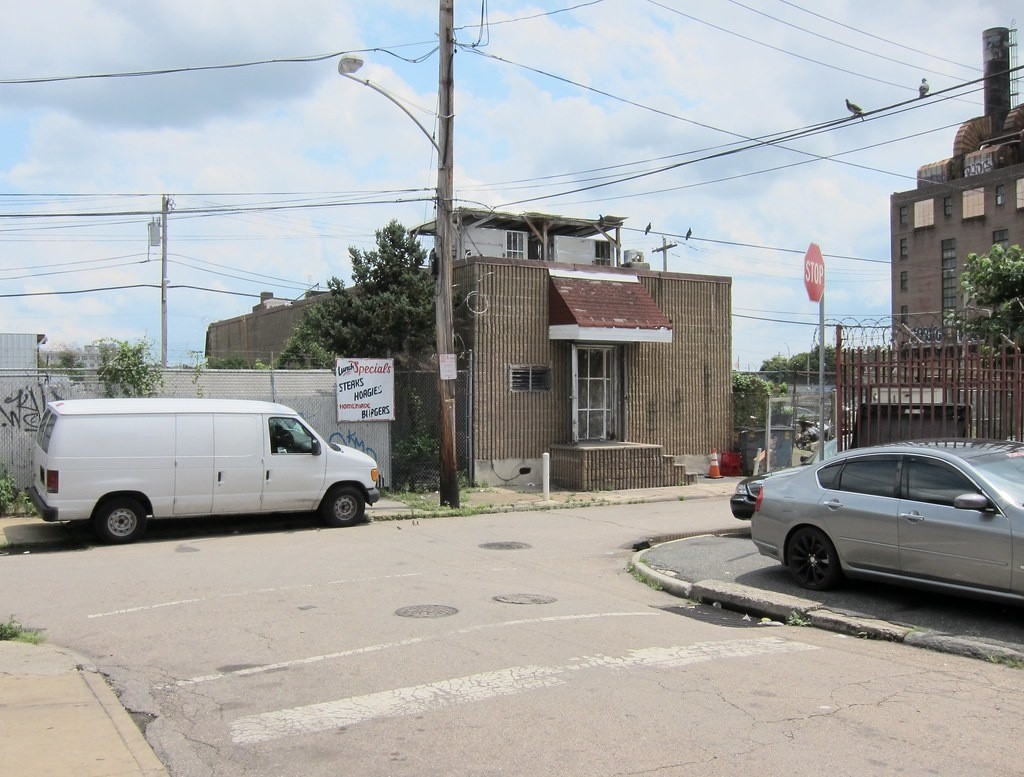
<box><xmin>22</xmin><ymin>398</ymin><xmax>383</xmax><ymax>546</ymax></box>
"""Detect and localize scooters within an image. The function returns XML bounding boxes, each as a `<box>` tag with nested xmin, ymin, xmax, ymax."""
<box><xmin>791</xmin><ymin>414</ymin><xmax>834</xmax><ymax>450</ymax></box>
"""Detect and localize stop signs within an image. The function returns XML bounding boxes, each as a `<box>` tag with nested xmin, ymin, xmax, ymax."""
<box><xmin>803</xmin><ymin>242</ymin><xmax>827</xmax><ymax>303</ymax></box>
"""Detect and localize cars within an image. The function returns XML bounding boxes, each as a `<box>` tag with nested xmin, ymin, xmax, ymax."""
<box><xmin>781</xmin><ymin>405</ymin><xmax>816</xmax><ymax>433</ymax></box>
<box><xmin>752</xmin><ymin>436</ymin><xmax>1024</xmax><ymax>615</ymax></box>
<box><xmin>730</xmin><ymin>434</ymin><xmax>856</xmax><ymax>521</ymax></box>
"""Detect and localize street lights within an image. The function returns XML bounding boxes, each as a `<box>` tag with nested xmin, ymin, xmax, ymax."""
<box><xmin>337</xmin><ymin>52</ymin><xmax>461</xmax><ymax>510</ymax></box>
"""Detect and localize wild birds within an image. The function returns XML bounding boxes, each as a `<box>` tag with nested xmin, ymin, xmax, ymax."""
<box><xmin>845</xmin><ymin>99</ymin><xmax>864</xmax><ymax>118</ymax></box>
<box><xmin>645</xmin><ymin>222</ymin><xmax>652</xmax><ymax>236</ymax></box>
<box><xmin>686</xmin><ymin>228</ymin><xmax>692</xmax><ymax>241</ymax></box>
<box><xmin>919</xmin><ymin>77</ymin><xmax>929</xmax><ymax>98</ymax></box>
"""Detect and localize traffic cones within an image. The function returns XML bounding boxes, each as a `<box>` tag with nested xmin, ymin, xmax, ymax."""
<box><xmin>704</xmin><ymin>447</ymin><xmax>725</xmax><ymax>479</ymax></box>
